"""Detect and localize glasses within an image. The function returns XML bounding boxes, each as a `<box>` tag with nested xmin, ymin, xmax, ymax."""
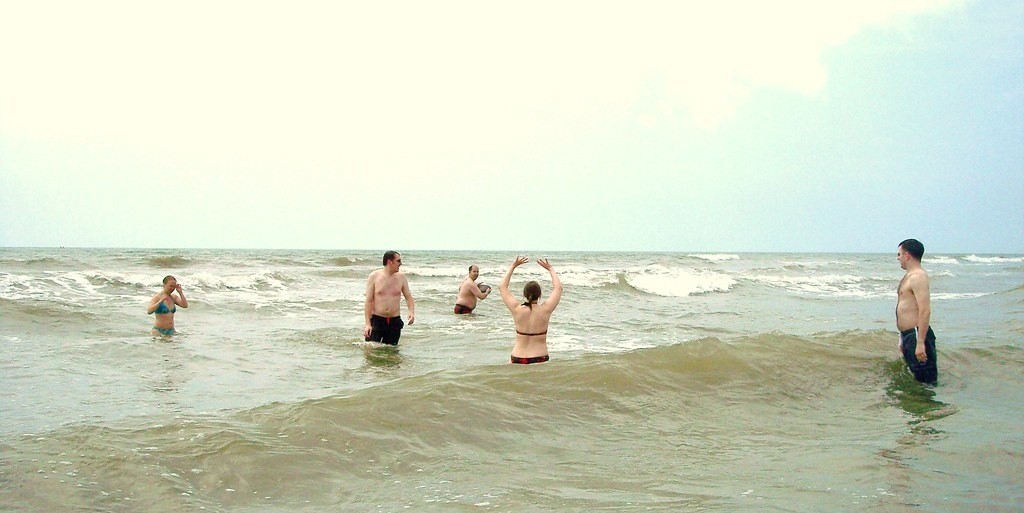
<box><xmin>898</xmin><ymin>252</ymin><xmax>906</xmax><ymax>256</ymax></box>
<box><xmin>393</xmin><ymin>259</ymin><xmax>400</xmax><ymax>262</ymax></box>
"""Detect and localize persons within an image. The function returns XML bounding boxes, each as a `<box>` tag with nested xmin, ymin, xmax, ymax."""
<box><xmin>499</xmin><ymin>256</ymin><xmax>562</xmax><ymax>364</ymax></box>
<box><xmin>895</xmin><ymin>239</ymin><xmax>938</xmax><ymax>387</ymax></box>
<box><xmin>453</xmin><ymin>265</ymin><xmax>490</xmax><ymax>314</ymax></box>
<box><xmin>146</xmin><ymin>275</ymin><xmax>188</xmax><ymax>336</ymax></box>
<box><xmin>363</xmin><ymin>251</ymin><xmax>414</xmax><ymax>345</ymax></box>
